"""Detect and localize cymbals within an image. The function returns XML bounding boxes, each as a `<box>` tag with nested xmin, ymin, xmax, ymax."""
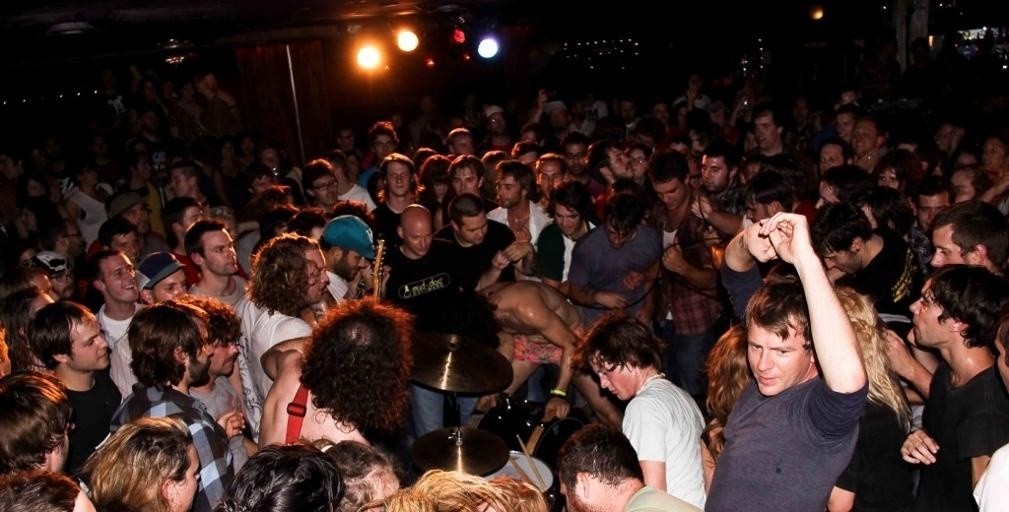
<box><xmin>410</xmin><ymin>427</ymin><xmax>510</xmax><ymax>476</ymax></box>
<box><xmin>408</xmin><ymin>334</ymin><xmax>514</xmax><ymax>394</ymax></box>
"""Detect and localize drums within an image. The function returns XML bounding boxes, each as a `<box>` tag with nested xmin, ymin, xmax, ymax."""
<box><xmin>485</xmin><ymin>452</ymin><xmax>555</xmax><ymax>494</ymax></box>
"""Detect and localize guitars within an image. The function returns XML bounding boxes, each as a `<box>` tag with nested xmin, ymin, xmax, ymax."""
<box><xmin>371</xmin><ymin>237</ymin><xmax>392</xmax><ymax>305</ymax></box>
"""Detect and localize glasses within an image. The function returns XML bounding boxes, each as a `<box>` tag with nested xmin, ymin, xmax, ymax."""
<box><xmin>384</xmin><ymin>172</ymin><xmax>411</xmax><ymax>179</ymax></box>
<box><xmin>311</xmin><ymin>178</ymin><xmax>339</xmax><ymax>190</ymax></box>
<box><xmin>49</xmin><ymin>272</ymin><xmax>76</xmax><ymax>283</ymax></box>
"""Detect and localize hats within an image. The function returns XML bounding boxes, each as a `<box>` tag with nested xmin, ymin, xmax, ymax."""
<box><xmin>322</xmin><ymin>214</ymin><xmax>375</xmax><ymax>261</ymax></box>
<box><xmin>483</xmin><ymin>105</ymin><xmax>504</xmax><ymax>116</ymax></box>
<box><xmin>17</xmin><ymin>249</ymin><xmax>71</xmax><ymax>271</ymax></box>
<box><xmin>134</xmin><ymin>250</ymin><xmax>187</xmax><ymax>288</ymax></box>
<box><xmin>109</xmin><ymin>193</ymin><xmax>152</xmax><ymax>216</ymax></box>
<box><xmin>544</xmin><ymin>101</ymin><xmax>566</xmax><ymax>111</ymax></box>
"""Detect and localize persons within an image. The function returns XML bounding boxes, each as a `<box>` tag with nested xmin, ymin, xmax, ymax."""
<box><xmin>1</xmin><ymin>71</ymin><xmax>1008</xmax><ymax>512</ymax></box>
